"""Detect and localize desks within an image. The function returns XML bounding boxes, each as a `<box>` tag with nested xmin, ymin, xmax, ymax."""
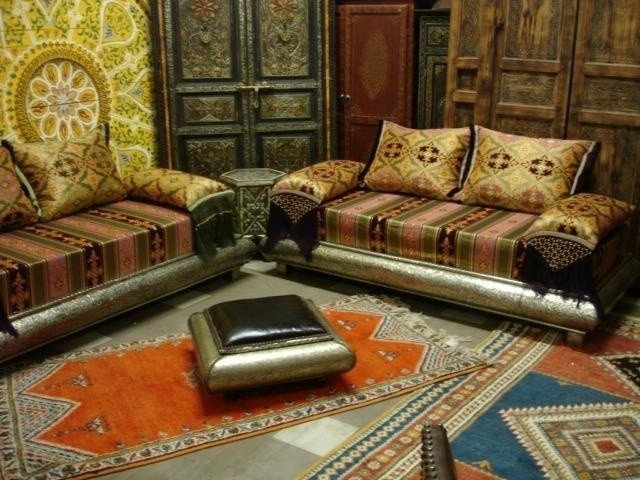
<box><xmin>219</xmin><ymin>167</ymin><xmax>288</xmax><ymax>262</ymax></box>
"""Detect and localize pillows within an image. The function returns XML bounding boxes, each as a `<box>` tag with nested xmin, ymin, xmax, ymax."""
<box><xmin>2</xmin><ymin>120</ymin><xmax>127</xmax><ymax>223</ymax></box>
<box><xmin>0</xmin><ymin>141</ymin><xmax>38</xmax><ymax>232</ymax></box>
<box><xmin>449</xmin><ymin>123</ymin><xmax>598</xmax><ymax>215</ymax></box>
<box><xmin>357</xmin><ymin>119</ymin><xmax>474</xmax><ymax>202</ymax></box>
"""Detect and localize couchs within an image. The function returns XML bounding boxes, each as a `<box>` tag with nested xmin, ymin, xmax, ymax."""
<box><xmin>185</xmin><ymin>291</ymin><xmax>358</xmax><ymax>402</ymax></box>
<box><xmin>256</xmin><ymin>158</ymin><xmax>640</xmax><ymax>349</ymax></box>
<box><xmin>0</xmin><ymin>167</ymin><xmax>256</xmax><ymax>366</ymax></box>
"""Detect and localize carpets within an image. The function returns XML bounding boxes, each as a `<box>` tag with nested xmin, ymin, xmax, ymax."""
<box><xmin>0</xmin><ymin>290</ymin><xmax>499</xmax><ymax>480</ymax></box>
<box><xmin>292</xmin><ymin>293</ymin><xmax>640</xmax><ymax>480</ymax></box>
